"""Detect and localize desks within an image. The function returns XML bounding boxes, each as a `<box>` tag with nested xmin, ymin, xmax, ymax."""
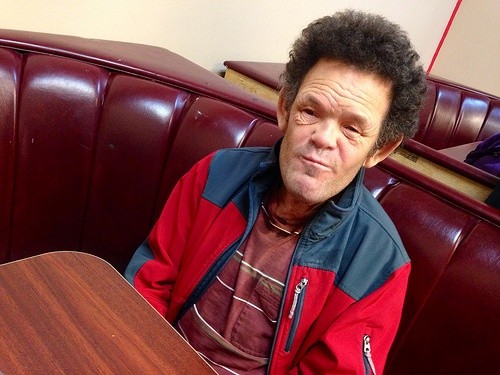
<box><xmin>0</xmin><ymin>250</ymin><xmax>219</xmax><ymax>375</ymax></box>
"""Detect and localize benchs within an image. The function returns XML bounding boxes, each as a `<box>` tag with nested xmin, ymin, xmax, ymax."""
<box><xmin>0</xmin><ymin>28</ymin><xmax>500</xmax><ymax>375</ymax></box>
<box><xmin>223</xmin><ymin>61</ymin><xmax>500</xmax><ymax>202</ymax></box>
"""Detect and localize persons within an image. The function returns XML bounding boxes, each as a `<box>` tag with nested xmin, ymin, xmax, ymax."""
<box><xmin>123</xmin><ymin>9</ymin><xmax>428</xmax><ymax>375</ymax></box>
